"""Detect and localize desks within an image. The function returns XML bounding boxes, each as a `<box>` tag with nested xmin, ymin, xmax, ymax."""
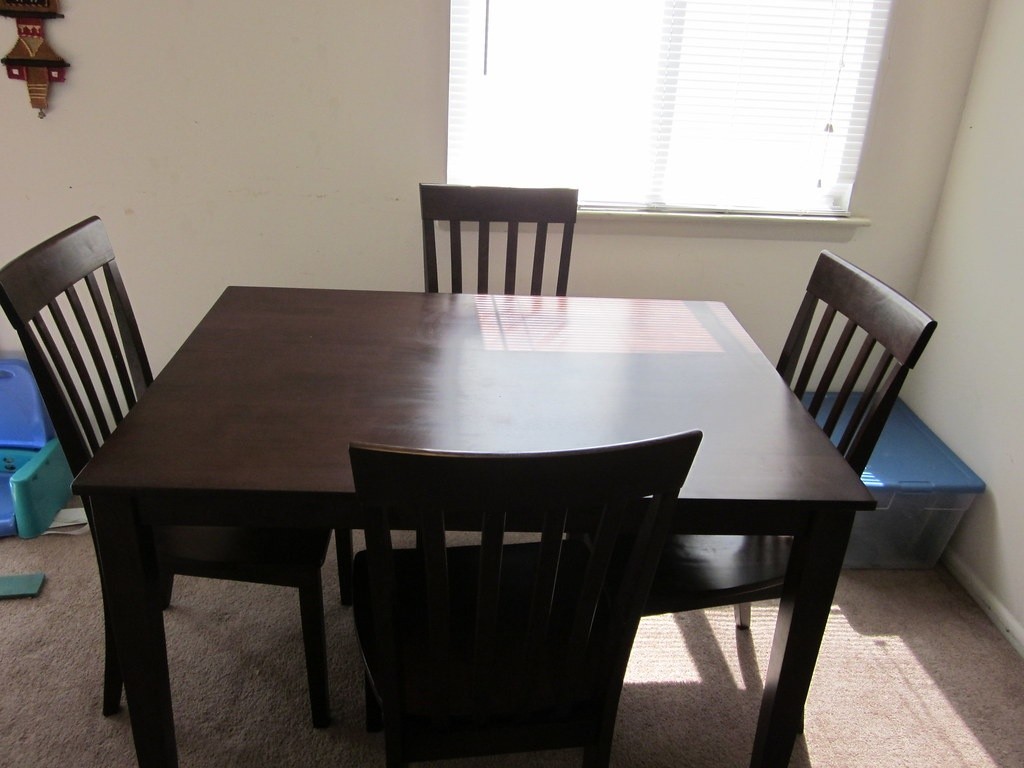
<box><xmin>73</xmin><ymin>285</ymin><xmax>876</xmax><ymax>768</ymax></box>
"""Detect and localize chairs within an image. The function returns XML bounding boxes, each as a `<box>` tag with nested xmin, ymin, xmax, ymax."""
<box><xmin>564</xmin><ymin>249</ymin><xmax>937</xmax><ymax>633</ymax></box>
<box><xmin>0</xmin><ymin>214</ymin><xmax>354</xmax><ymax>729</ymax></box>
<box><xmin>344</xmin><ymin>429</ymin><xmax>701</xmax><ymax>768</ymax></box>
<box><xmin>0</xmin><ymin>357</ymin><xmax>72</xmax><ymax>540</ymax></box>
<box><xmin>417</xmin><ymin>181</ymin><xmax>581</xmax><ymax>298</ymax></box>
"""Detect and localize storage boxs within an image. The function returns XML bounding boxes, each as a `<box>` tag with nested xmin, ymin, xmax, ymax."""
<box><xmin>796</xmin><ymin>390</ymin><xmax>983</xmax><ymax>574</ymax></box>
